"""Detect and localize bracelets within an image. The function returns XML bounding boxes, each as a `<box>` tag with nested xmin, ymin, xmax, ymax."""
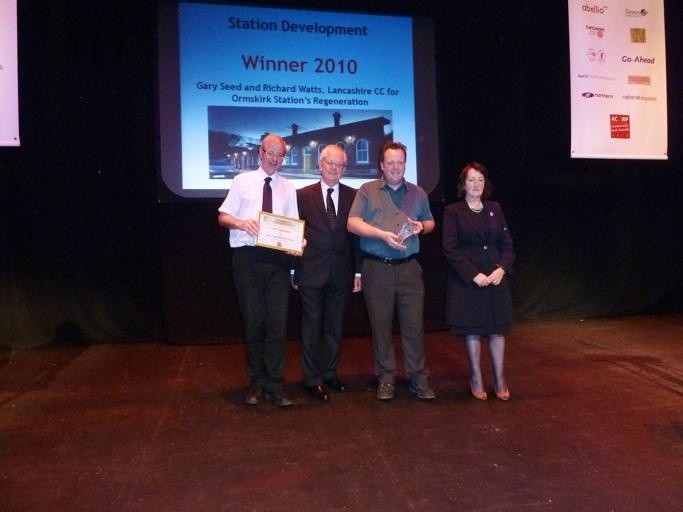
<box><xmin>419</xmin><ymin>221</ymin><xmax>425</xmax><ymax>233</ymax></box>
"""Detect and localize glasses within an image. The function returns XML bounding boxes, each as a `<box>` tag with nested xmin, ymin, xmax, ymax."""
<box><xmin>262</xmin><ymin>148</ymin><xmax>282</xmax><ymax>159</ymax></box>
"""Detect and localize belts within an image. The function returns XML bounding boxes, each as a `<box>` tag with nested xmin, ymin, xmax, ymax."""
<box><xmin>365</xmin><ymin>253</ymin><xmax>414</xmax><ymax>266</ymax></box>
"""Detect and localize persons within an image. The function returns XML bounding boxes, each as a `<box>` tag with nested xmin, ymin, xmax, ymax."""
<box><xmin>345</xmin><ymin>141</ymin><xmax>436</xmax><ymax>402</ymax></box>
<box><xmin>289</xmin><ymin>144</ymin><xmax>363</xmax><ymax>404</ymax></box>
<box><xmin>440</xmin><ymin>159</ymin><xmax>516</xmax><ymax>402</ymax></box>
<box><xmin>217</xmin><ymin>134</ymin><xmax>309</xmax><ymax>407</ymax></box>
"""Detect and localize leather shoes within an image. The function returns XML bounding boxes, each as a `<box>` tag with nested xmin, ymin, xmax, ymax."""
<box><xmin>376</xmin><ymin>383</ymin><xmax>395</xmax><ymax>400</ymax></box>
<box><xmin>308</xmin><ymin>384</ymin><xmax>330</xmax><ymax>401</ymax></box>
<box><xmin>467</xmin><ymin>375</ymin><xmax>487</xmax><ymax>401</ymax></box>
<box><xmin>245</xmin><ymin>384</ymin><xmax>264</xmax><ymax>404</ymax></box>
<box><xmin>268</xmin><ymin>389</ymin><xmax>292</xmax><ymax>407</ymax></box>
<box><xmin>494</xmin><ymin>383</ymin><xmax>509</xmax><ymax>401</ymax></box>
<box><xmin>408</xmin><ymin>382</ymin><xmax>435</xmax><ymax>399</ymax></box>
<box><xmin>325</xmin><ymin>378</ymin><xmax>348</xmax><ymax>392</ymax></box>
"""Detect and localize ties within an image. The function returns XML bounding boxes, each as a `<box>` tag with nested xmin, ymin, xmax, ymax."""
<box><xmin>262</xmin><ymin>177</ymin><xmax>271</xmax><ymax>213</ymax></box>
<box><xmin>326</xmin><ymin>189</ymin><xmax>336</xmax><ymax>226</ymax></box>
